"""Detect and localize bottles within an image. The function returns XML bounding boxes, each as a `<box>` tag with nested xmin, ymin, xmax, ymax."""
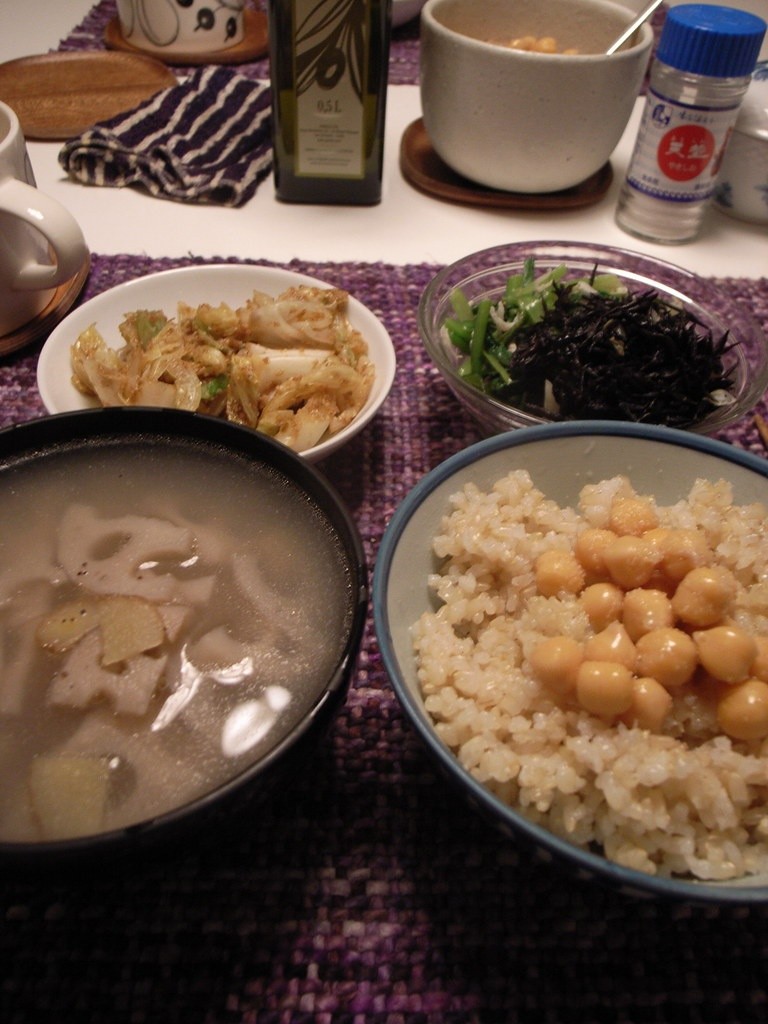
<box><xmin>269</xmin><ymin>0</ymin><xmax>393</xmax><ymax>206</ymax></box>
<box><xmin>615</xmin><ymin>4</ymin><xmax>767</xmax><ymax>246</ymax></box>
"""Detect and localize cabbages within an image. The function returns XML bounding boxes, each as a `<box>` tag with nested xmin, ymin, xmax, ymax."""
<box><xmin>68</xmin><ymin>285</ymin><xmax>376</xmax><ymax>454</ymax></box>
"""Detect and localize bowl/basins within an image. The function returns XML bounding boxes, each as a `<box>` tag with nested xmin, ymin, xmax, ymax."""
<box><xmin>420</xmin><ymin>0</ymin><xmax>652</xmax><ymax>193</ymax></box>
<box><xmin>0</xmin><ymin>405</ymin><xmax>367</xmax><ymax>849</ymax></box>
<box><xmin>416</xmin><ymin>238</ymin><xmax>767</xmax><ymax>433</ymax></box>
<box><xmin>371</xmin><ymin>417</ymin><xmax>767</xmax><ymax>903</ymax></box>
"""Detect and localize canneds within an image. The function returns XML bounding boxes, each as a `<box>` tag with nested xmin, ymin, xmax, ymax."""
<box><xmin>614</xmin><ymin>2</ymin><xmax>767</xmax><ymax>244</ymax></box>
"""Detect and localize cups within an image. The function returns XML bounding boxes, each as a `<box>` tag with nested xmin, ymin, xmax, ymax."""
<box><xmin>712</xmin><ymin>60</ymin><xmax>767</xmax><ymax>225</ymax></box>
<box><xmin>116</xmin><ymin>0</ymin><xmax>245</xmax><ymax>54</ymax></box>
<box><xmin>0</xmin><ymin>98</ymin><xmax>90</xmax><ymax>355</ymax></box>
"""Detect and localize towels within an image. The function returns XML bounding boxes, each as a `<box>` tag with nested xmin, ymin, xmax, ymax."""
<box><xmin>56</xmin><ymin>63</ymin><xmax>276</xmax><ymax>208</ymax></box>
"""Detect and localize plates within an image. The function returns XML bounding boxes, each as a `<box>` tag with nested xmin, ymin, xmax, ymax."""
<box><xmin>37</xmin><ymin>262</ymin><xmax>397</xmax><ymax>459</ymax></box>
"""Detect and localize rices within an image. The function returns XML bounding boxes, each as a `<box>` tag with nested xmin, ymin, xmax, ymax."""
<box><xmin>407</xmin><ymin>468</ymin><xmax>768</xmax><ymax>883</ymax></box>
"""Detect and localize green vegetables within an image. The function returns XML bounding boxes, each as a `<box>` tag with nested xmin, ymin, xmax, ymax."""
<box><xmin>442</xmin><ymin>254</ymin><xmax>628</xmax><ymax>394</ymax></box>
